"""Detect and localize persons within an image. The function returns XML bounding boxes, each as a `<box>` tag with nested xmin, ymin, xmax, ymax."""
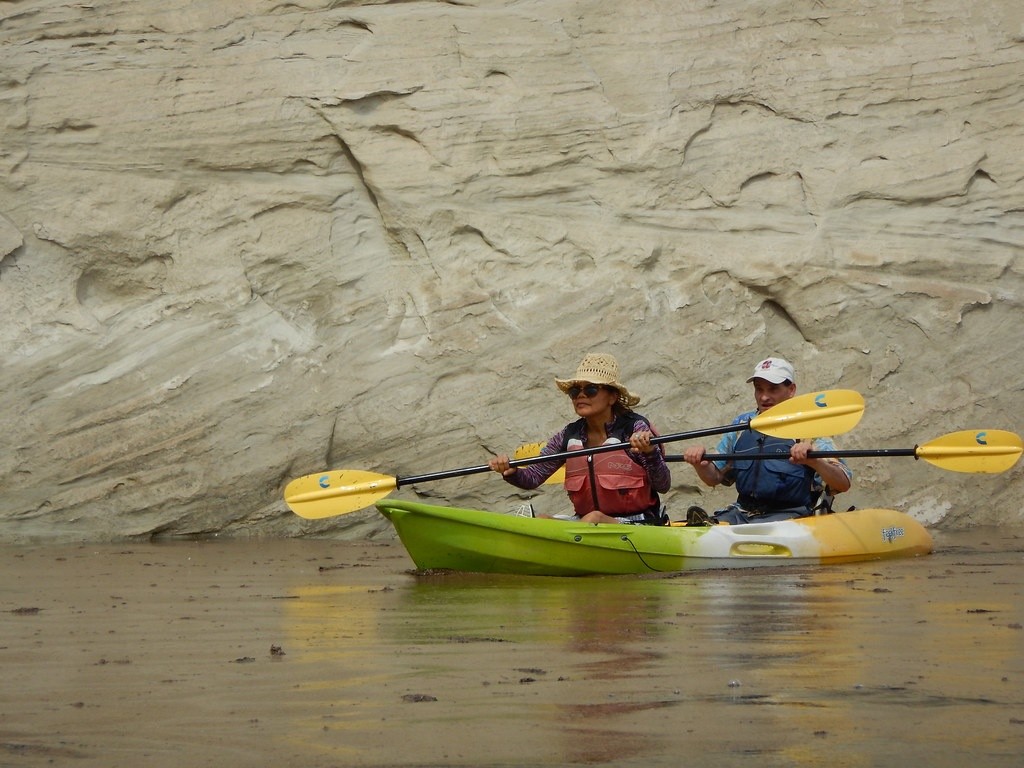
<box><xmin>488</xmin><ymin>352</ymin><xmax>671</xmax><ymax>524</ymax></box>
<box><xmin>681</xmin><ymin>357</ymin><xmax>853</xmax><ymax>526</ymax></box>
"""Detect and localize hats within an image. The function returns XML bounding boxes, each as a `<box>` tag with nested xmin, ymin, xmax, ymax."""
<box><xmin>554</xmin><ymin>353</ymin><xmax>640</xmax><ymax>406</ymax></box>
<box><xmin>746</xmin><ymin>358</ymin><xmax>796</xmax><ymax>385</ymax></box>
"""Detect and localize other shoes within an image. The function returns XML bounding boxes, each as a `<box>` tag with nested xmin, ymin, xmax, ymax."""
<box><xmin>687</xmin><ymin>506</ymin><xmax>710</xmax><ymax>526</ymax></box>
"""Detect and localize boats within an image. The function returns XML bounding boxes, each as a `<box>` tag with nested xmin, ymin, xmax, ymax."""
<box><xmin>374</xmin><ymin>497</ymin><xmax>935</xmax><ymax>575</ymax></box>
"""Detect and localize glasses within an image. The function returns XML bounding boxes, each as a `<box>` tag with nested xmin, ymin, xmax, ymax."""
<box><xmin>568</xmin><ymin>383</ymin><xmax>613</xmax><ymax>400</ymax></box>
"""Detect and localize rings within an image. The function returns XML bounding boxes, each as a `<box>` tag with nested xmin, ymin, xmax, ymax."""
<box><xmin>635</xmin><ymin>434</ymin><xmax>639</xmax><ymax>437</ymax></box>
<box><xmin>641</xmin><ymin>434</ymin><xmax>644</xmax><ymax>437</ymax></box>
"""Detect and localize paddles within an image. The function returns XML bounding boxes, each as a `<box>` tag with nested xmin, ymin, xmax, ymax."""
<box><xmin>280</xmin><ymin>386</ymin><xmax>869</xmax><ymax>525</ymax></box>
<box><xmin>512</xmin><ymin>428</ymin><xmax>1024</xmax><ymax>486</ymax></box>
